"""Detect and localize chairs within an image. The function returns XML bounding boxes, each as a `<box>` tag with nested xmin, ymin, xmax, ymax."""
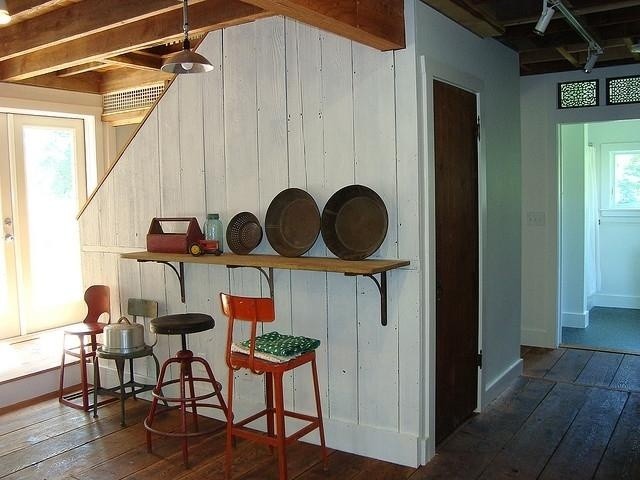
<box><xmin>219</xmin><ymin>294</ymin><xmax>328</xmax><ymax>479</ymax></box>
<box><xmin>94</xmin><ymin>298</ymin><xmax>169</xmax><ymax>426</ymax></box>
<box><xmin>59</xmin><ymin>285</ymin><xmax>127</xmax><ymax>412</ymax></box>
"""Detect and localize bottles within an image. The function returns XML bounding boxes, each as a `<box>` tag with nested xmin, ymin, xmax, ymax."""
<box><xmin>202</xmin><ymin>213</ymin><xmax>225</xmax><ymax>256</ymax></box>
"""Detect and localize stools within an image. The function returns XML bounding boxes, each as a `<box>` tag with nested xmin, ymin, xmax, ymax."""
<box><xmin>144</xmin><ymin>312</ymin><xmax>235</xmax><ymax>468</ymax></box>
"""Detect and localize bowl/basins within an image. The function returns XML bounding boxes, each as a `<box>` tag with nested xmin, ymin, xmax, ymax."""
<box><xmin>264</xmin><ymin>187</ymin><xmax>321</xmax><ymax>257</ymax></box>
<box><xmin>321</xmin><ymin>184</ymin><xmax>388</xmax><ymax>261</ymax></box>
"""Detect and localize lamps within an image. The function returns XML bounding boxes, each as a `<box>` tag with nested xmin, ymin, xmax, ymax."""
<box><xmin>0</xmin><ymin>0</ymin><xmax>11</xmax><ymax>25</ymax></box>
<box><xmin>161</xmin><ymin>0</ymin><xmax>214</xmax><ymax>74</ymax></box>
<box><xmin>533</xmin><ymin>0</ymin><xmax>600</xmax><ymax>73</ymax></box>
